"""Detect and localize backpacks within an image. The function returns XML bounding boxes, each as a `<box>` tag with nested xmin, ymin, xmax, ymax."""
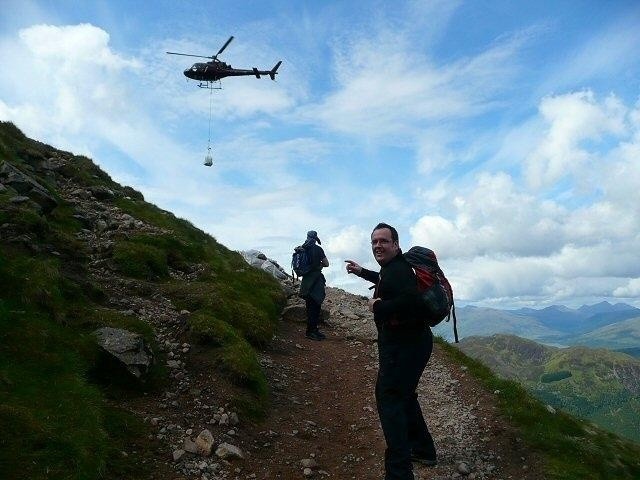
<box><xmin>291</xmin><ymin>245</ymin><xmax>319</xmax><ymax>284</ymax></box>
<box><xmin>402</xmin><ymin>246</ymin><xmax>459</xmax><ymax>342</ymax></box>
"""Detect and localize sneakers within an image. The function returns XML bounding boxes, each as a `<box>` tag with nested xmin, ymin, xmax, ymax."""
<box><xmin>409</xmin><ymin>448</ymin><xmax>437</xmax><ymax>466</ymax></box>
<box><xmin>305</xmin><ymin>331</ymin><xmax>321</xmax><ymax>340</ymax></box>
<box><xmin>314</xmin><ymin>330</ymin><xmax>326</xmax><ymax>338</ymax></box>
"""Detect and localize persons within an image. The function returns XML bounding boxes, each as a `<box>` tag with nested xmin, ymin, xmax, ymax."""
<box><xmin>345</xmin><ymin>221</ymin><xmax>438</xmax><ymax>477</ymax></box>
<box><xmin>301</xmin><ymin>229</ymin><xmax>331</xmax><ymax>341</ymax></box>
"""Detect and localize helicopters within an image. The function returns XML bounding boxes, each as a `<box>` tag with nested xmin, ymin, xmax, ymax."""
<box><xmin>165</xmin><ymin>34</ymin><xmax>284</xmax><ymax>92</ymax></box>
